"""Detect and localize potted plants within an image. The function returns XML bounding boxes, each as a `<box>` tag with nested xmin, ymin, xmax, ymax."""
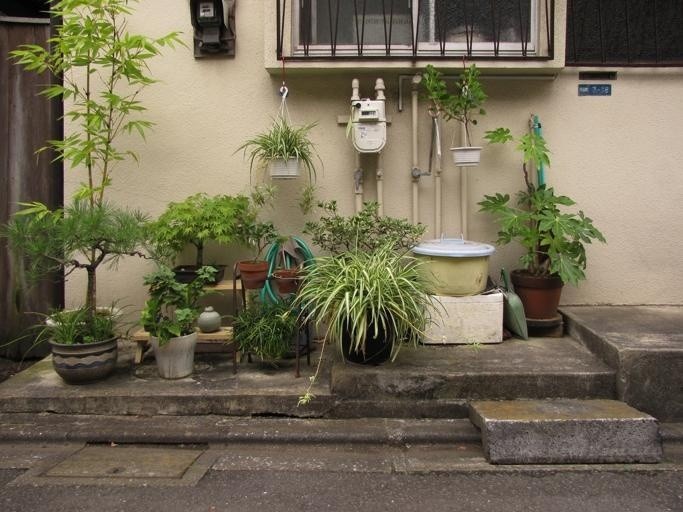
<box><xmin>477</xmin><ymin>112</ymin><xmax>607</xmax><ymax>319</ymax></box>
<box><xmin>1</xmin><ymin>199</ymin><xmax>158</xmax><ymax>382</ymax></box>
<box><xmin>423</xmin><ymin>63</ymin><xmax>489</xmax><ymax>167</ymax></box>
<box><xmin>221</xmin><ymin>289</ymin><xmax>307</xmax><ymax>369</ymax></box>
<box><xmin>234</xmin><ymin>201</ymin><xmax>429</xmax><ymax>296</ymax></box>
<box><xmin>147</xmin><ymin>192</ymin><xmax>259</xmax><ymax>286</ymax></box>
<box><xmin>6</xmin><ymin>1</ymin><xmax>191</xmax><ymax>330</ymax></box>
<box><xmin>232</xmin><ymin>87</ymin><xmax>324</xmax><ymax>214</ymax></box>
<box><xmin>141</xmin><ymin>265</ymin><xmax>219</xmax><ymax>380</ymax></box>
<box><xmin>277</xmin><ymin>228</ymin><xmax>450</xmax><ymax>406</ymax></box>
